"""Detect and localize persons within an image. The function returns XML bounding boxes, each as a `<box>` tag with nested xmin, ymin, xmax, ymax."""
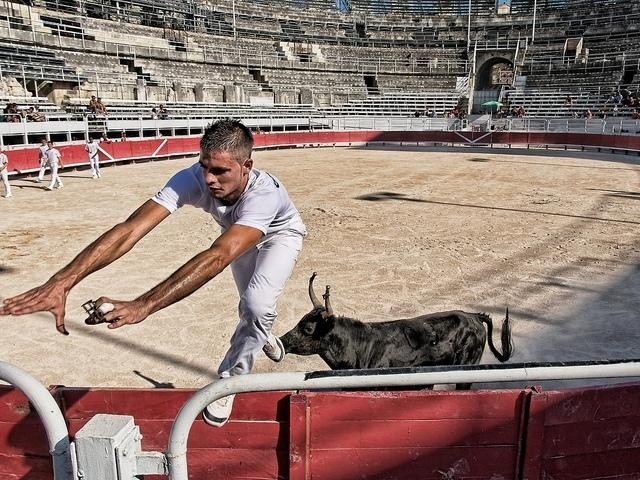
<box><xmin>564</xmin><ymin>88</ymin><xmax>640</xmax><ymax>121</ymax></box>
<box><xmin>1</xmin><ymin>115</ymin><xmax>308</xmax><ymax>431</ymax></box>
<box><xmin>1</xmin><ymin>93</ymin><xmax>171</xmax><ymax>198</ymax></box>
<box><xmin>413</xmin><ymin>104</ymin><xmax>526</xmax><ymax>132</ymax></box>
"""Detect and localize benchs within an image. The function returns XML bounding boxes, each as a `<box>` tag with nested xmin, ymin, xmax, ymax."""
<box><xmin>0</xmin><ymin>0</ymin><xmax>640</xmax><ymax>145</ymax></box>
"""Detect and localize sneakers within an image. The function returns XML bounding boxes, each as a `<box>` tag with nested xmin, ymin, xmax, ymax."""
<box><xmin>262</xmin><ymin>333</ymin><xmax>285</xmax><ymax>363</ymax></box>
<box><xmin>202</xmin><ymin>370</ymin><xmax>236</xmax><ymax>427</ymax></box>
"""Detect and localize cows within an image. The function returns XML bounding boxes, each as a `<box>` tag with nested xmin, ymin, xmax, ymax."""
<box><xmin>279</xmin><ymin>272</ymin><xmax>515</xmax><ymax>391</ymax></box>
